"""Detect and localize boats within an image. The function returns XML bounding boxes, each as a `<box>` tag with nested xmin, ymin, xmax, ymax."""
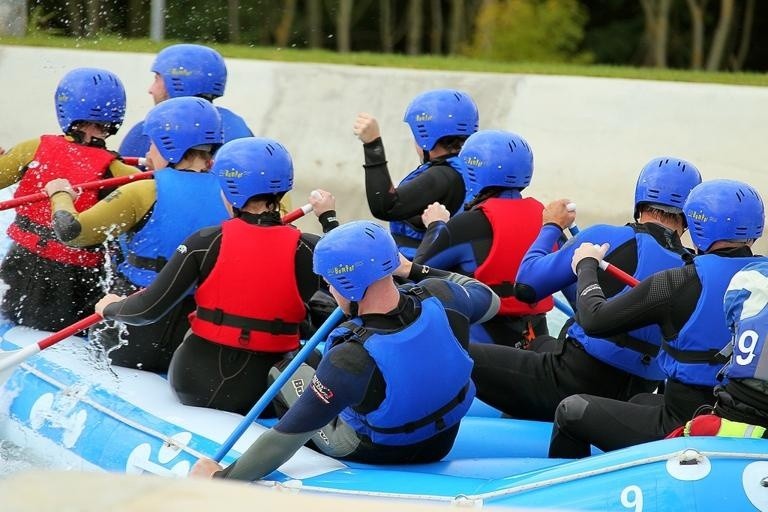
<box><xmin>0</xmin><ymin>198</ymin><xmax>767</xmax><ymax>512</ymax></box>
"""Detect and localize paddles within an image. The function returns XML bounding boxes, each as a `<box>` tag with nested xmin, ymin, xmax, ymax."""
<box><xmin>1</xmin><ymin>191</ymin><xmax>323</xmax><ymax>378</ymax></box>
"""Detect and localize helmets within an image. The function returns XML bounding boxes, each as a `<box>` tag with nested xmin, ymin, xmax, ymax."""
<box><xmin>312</xmin><ymin>221</ymin><xmax>403</xmax><ymax>303</ymax></box>
<box><xmin>460</xmin><ymin>129</ymin><xmax>535</xmax><ymax>195</ymax></box>
<box><xmin>55</xmin><ymin>67</ymin><xmax>127</xmax><ymax>137</ymax></box>
<box><xmin>403</xmin><ymin>89</ymin><xmax>479</xmax><ymax>151</ymax></box>
<box><xmin>143</xmin><ymin>96</ymin><xmax>225</xmax><ymax>164</ymax></box>
<box><xmin>151</xmin><ymin>43</ymin><xmax>228</xmax><ymax>99</ymax></box>
<box><xmin>634</xmin><ymin>157</ymin><xmax>702</xmax><ymax>221</ymax></box>
<box><xmin>683</xmin><ymin>179</ymin><xmax>765</xmax><ymax>251</ymax></box>
<box><xmin>212</xmin><ymin>137</ymin><xmax>296</xmax><ymax>211</ymax></box>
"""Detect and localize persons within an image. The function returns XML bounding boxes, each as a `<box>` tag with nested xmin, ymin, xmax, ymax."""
<box><xmin>708</xmin><ymin>259</ymin><xmax>768</xmax><ymax>438</ymax></box>
<box><xmin>463</xmin><ymin>156</ymin><xmax>702</xmax><ymax>421</ymax></box>
<box><xmin>116</xmin><ymin>44</ymin><xmax>249</xmax><ymax>158</ymax></box>
<box><xmin>93</xmin><ymin>133</ymin><xmax>319</xmax><ymax>418</ymax></box>
<box><xmin>546</xmin><ymin>176</ymin><xmax>768</xmax><ymax>462</ymax></box>
<box><xmin>188</xmin><ymin>219</ymin><xmax>503</xmax><ymax>485</ymax></box>
<box><xmin>412</xmin><ymin>128</ymin><xmax>550</xmax><ymax>347</ymax></box>
<box><xmin>44</xmin><ymin>95</ymin><xmax>231</xmax><ymax>377</ymax></box>
<box><xmin>347</xmin><ymin>87</ymin><xmax>480</xmax><ymax>261</ymax></box>
<box><xmin>0</xmin><ymin>65</ymin><xmax>143</xmax><ymax>339</ymax></box>
